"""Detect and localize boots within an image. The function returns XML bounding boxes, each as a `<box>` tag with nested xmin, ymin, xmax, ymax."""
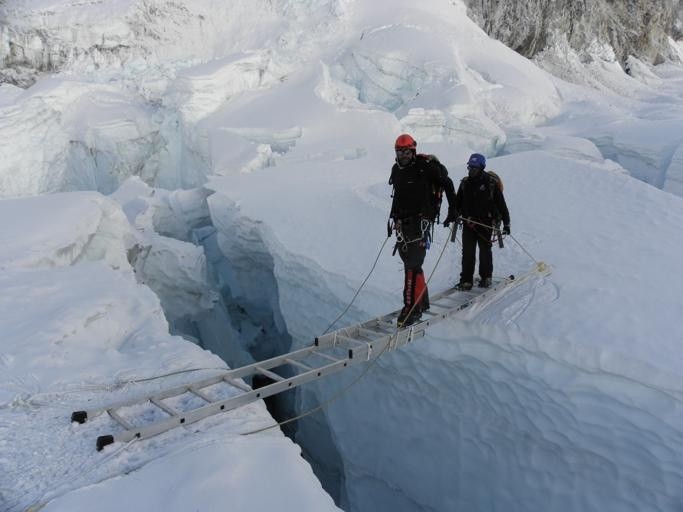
<box><xmin>398</xmin><ymin>269</ymin><xmax>430</xmax><ymax>326</ymax></box>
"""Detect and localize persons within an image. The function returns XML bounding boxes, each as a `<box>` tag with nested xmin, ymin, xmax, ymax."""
<box><xmin>386</xmin><ymin>134</ymin><xmax>456</xmax><ymax>327</ymax></box>
<box><xmin>443</xmin><ymin>153</ymin><xmax>511</xmax><ymax>290</ymax></box>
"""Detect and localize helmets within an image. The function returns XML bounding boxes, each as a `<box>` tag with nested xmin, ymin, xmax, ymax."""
<box><xmin>467</xmin><ymin>153</ymin><xmax>486</xmax><ymax>168</ymax></box>
<box><xmin>395</xmin><ymin>134</ymin><xmax>416</xmax><ymax>153</ymax></box>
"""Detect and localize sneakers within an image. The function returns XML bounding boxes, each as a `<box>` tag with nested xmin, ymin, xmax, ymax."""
<box><xmin>478</xmin><ymin>277</ymin><xmax>492</xmax><ymax>287</ymax></box>
<box><xmin>456</xmin><ymin>275</ymin><xmax>473</xmax><ymax>290</ymax></box>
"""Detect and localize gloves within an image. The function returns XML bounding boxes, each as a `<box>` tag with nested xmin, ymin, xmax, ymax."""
<box><xmin>444</xmin><ymin>205</ymin><xmax>457</xmax><ymax>228</ymax></box>
<box><xmin>503</xmin><ymin>225</ymin><xmax>510</xmax><ymax>235</ymax></box>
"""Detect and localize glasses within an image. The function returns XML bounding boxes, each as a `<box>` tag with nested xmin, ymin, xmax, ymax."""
<box><xmin>467</xmin><ymin>166</ymin><xmax>477</xmax><ymax>170</ymax></box>
<box><xmin>397</xmin><ymin>150</ymin><xmax>412</xmax><ymax>156</ymax></box>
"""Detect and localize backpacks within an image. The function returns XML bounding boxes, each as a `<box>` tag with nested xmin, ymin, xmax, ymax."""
<box><xmin>395</xmin><ymin>154</ymin><xmax>448</xmax><ymax>216</ymax></box>
<box><xmin>461</xmin><ymin>171</ymin><xmax>503</xmax><ymax>201</ymax></box>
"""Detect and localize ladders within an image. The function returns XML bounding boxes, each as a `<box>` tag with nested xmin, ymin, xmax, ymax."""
<box><xmin>71</xmin><ymin>274</ymin><xmax>515</xmax><ymax>452</ymax></box>
